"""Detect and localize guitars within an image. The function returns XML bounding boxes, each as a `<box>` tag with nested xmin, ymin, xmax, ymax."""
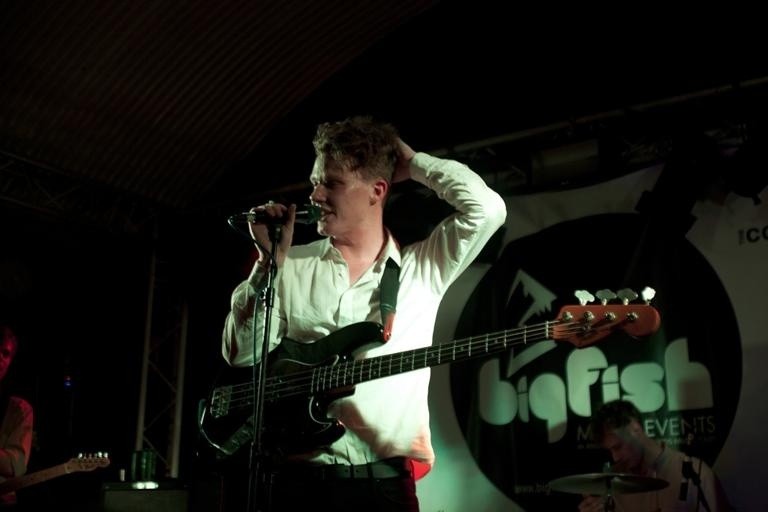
<box><xmin>195</xmin><ymin>287</ymin><xmax>661</xmax><ymax>467</ymax></box>
<box><xmin>0</xmin><ymin>451</ymin><xmax>110</xmax><ymax>498</ymax></box>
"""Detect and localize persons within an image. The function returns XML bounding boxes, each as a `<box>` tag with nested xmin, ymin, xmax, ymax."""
<box><xmin>1</xmin><ymin>320</ymin><xmax>33</xmax><ymax>510</ymax></box>
<box><xmin>216</xmin><ymin>117</ymin><xmax>507</xmax><ymax>512</ymax></box>
<box><xmin>578</xmin><ymin>399</ymin><xmax>728</xmax><ymax>511</ymax></box>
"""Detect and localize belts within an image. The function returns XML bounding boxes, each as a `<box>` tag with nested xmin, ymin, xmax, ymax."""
<box><xmin>288</xmin><ymin>455</ymin><xmax>405</xmax><ymax>480</ymax></box>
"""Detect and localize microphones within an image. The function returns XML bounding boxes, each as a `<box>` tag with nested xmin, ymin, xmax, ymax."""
<box><xmin>231</xmin><ymin>203</ymin><xmax>321</xmax><ymax>225</ymax></box>
<box><xmin>679</xmin><ymin>450</ymin><xmax>692</xmax><ymax>502</ymax></box>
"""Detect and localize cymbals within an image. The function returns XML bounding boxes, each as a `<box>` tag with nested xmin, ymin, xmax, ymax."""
<box><xmin>547</xmin><ymin>471</ymin><xmax>669</xmax><ymax>497</ymax></box>
